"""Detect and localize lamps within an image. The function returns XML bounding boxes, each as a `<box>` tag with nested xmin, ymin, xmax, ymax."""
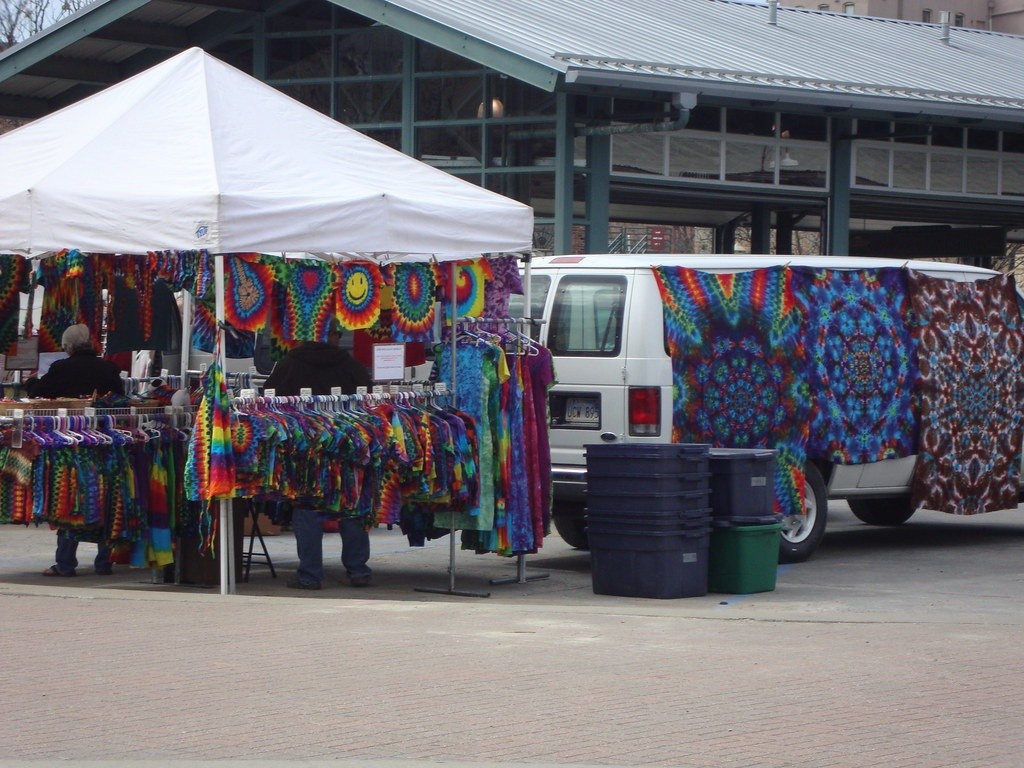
<box><xmin>780</xmin><ymin>147</ymin><xmax>799</xmax><ymax>167</ymax></box>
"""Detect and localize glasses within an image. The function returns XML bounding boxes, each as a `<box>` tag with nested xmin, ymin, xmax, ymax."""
<box><xmin>334</xmin><ymin>331</ymin><xmax>343</xmax><ymax>339</ymax></box>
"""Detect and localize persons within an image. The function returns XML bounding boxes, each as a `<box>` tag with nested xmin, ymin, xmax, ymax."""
<box><xmin>25</xmin><ymin>323</ymin><xmax>124</xmax><ymax>577</ymax></box>
<box><xmin>263</xmin><ymin>319</ymin><xmax>372</xmax><ymax>588</ymax></box>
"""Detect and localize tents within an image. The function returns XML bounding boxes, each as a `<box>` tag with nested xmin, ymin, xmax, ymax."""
<box><xmin>0</xmin><ymin>44</ymin><xmax>534</xmax><ymax>596</ymax></box>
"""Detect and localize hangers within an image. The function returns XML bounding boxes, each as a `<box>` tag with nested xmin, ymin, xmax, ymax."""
<box><xmin>121</xmin><ymin>375</ymin><xmax>182</xmax><ymax>399</ymax></box>
<box><xmin>432</xmin><ymin>315</ymin><xmax>541</xmax><ymax>357</ymax></box>
<box><xmin>2</xmin><ymin>411</ymin><xmax>199</xmax><ymax>447</ymax></box>
<box><xmin>231</xmin><ymin>371</ymin><xmax>450</xmax><ymax>418</ymax></box>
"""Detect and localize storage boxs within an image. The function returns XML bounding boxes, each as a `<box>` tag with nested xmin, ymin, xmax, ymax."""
<box><xmin>582</xmin><ymin>442</ymin><xmax>784</xmax><ymax>599</ymax></box>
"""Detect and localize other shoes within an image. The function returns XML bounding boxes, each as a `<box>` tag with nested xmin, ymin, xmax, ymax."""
<box><xmin>285</xmin><ymin>571</ymin><xmax>321</xmax><ymax>589</ymax></box>
<box><xmin>43</xmin><ymin>564</ymin><xmax>75</xmax><ymax>578</ymax></box>
<box><xmin>95</xmin><ymin>564</ymin><xmax>113</xmax><ymax>576</ymax></box>
<box><xmin>351</xmin><ymin>574</ymin><xmax>371</xmax><ymax>587</ymax></box>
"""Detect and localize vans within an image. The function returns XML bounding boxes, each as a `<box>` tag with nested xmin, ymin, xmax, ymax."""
<box><xmin>500</xmin><ymin>251</ymin><xmax>1024</xmax><ymax>552</ymax></box>
